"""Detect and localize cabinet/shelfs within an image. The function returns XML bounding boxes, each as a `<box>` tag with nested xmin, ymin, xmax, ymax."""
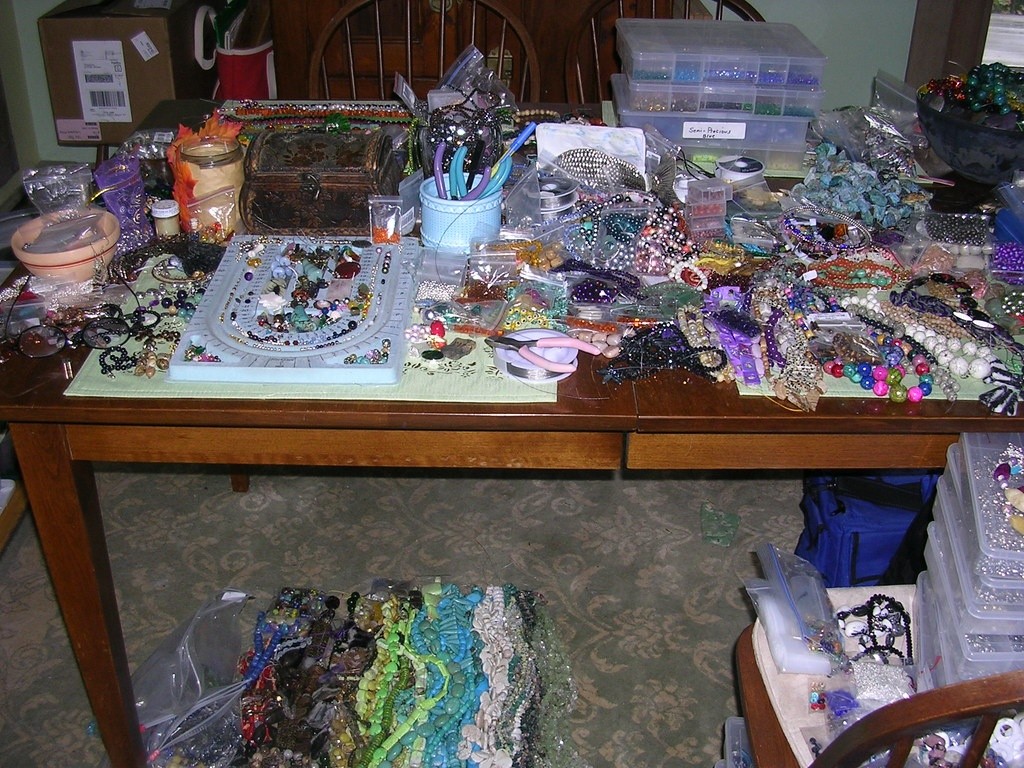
<box><xmin>269</xmin><ymin>0</ymin><xmax>674</xmax><ymax>103</ymax></box>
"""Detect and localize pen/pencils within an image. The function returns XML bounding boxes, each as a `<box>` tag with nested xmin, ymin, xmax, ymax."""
<box><xmin>466</xmin><ymin>141</ymin><xmax>486</xmax><ymax>191</ymax></box>
<box><xmin>490</xmin><ymin>121</ymin><xmax>536</xmax><ymax>179</ymax></box>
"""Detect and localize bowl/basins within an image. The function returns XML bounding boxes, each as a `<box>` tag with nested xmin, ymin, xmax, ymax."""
<box><xmin>10</xmin><ymin>209</ymin><xmax>121</xmax><ymax>280</ymax></box>
<box><xmin>914</xmin><ymin>83</ymin><xmax>1024</xmax><ymax>186</ymax></box>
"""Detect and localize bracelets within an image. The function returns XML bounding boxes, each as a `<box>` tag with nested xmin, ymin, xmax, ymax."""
<box><xmin>512</xmin><ymin>109</ymin><xmax>560</xmax><ymax>126</ymax></box>
<box><xmin>928</xmin><ymin>62</ymin><xmax>1024</xmax><ymax>116</ymax></box>
<box><xmin>33</xmin><ymin>256</ymin><xmax>215</xmax><ymax>381</ymax></box>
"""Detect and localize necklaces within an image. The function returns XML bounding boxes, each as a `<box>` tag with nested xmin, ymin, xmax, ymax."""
<box><xmin>539</xmin><ymin>193</ymin><xmax>1024</xmax><ymax>414</ymax></box>
<box><xmin>838</xmin><ymin>593</ymin><xmax>914</xmax><ymax>672</ymax></box>
<box><xmin>205</xmin><ymin>98</ymin><xmax>413</xmax><ymax>132</ymax></box>
<box><xmin>226</xmin><ymin>587</ymin><xmax>580</xmax><ymax>768</ymax></box>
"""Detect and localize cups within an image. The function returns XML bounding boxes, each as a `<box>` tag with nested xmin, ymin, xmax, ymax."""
<box><xmin>419</xmin><ymin>172</ymin><xmax>504</xmax><ymax>252</ymax></box>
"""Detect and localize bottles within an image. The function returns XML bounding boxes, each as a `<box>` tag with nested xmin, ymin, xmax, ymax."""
<box><xmin>151</xmin><ymin>200</ymin><xmax>180</xmax><ymax>236</ymax></box>
<box><xmin>175</xmin><ymin>134</ymin><xmax>246</xmax><ymax>224</ymax></box>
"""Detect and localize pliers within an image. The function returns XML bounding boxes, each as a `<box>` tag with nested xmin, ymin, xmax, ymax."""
<box><xmin>434</xmin><ymin>142</ymin><xmax>513</xmax><ymax>201</ymax></box>
<box><xmin>484</xmin><ymin>335</ymin><xmax>601</xmax><ymax>373</ymax></box>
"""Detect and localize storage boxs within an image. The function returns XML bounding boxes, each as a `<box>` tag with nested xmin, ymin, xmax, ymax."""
<box><xmin>238</xmin><ymin>127</ymin><xmax>400</xmax><ymax>236</ymax></box>
<box><xmin>715</xmin><ymin>717</ymin><xmax>756</xmax><ymax>768</ymax></box>
<box><xmin>903</xmin><ymin>427</ymin><xmax>1024</xmax><ymax>708</ymax></box>
<box><xmin>38</xmin><ymin>3</ymin><xmax>176</xmax><ymax>148</ymax></box>
<box><xmin>607</xmin><ymin>19</ymin><xmax>828</xmax><ymax>240</ymax></box>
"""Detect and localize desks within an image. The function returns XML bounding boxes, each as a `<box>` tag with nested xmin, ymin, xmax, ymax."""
<box><xmin>0</xmin><ymin>149</ymin><xmax>1024</xmax><ymax>768</ymax></box>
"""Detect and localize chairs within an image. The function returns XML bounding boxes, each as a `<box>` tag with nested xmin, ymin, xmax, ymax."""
<box><xmin>309</xmin><ymin>1</ymin><xmax>544</xmax><ymax>103</ymax></box>
<box><xmin>733</xmin><ymin>581</ymin><xmax>1024</xmax><ymax>768</ymax></box>
<box><xmin>564</xmin><ymin>0</ymin><xmax>766</xmax><ymax>105</ymax></box>
